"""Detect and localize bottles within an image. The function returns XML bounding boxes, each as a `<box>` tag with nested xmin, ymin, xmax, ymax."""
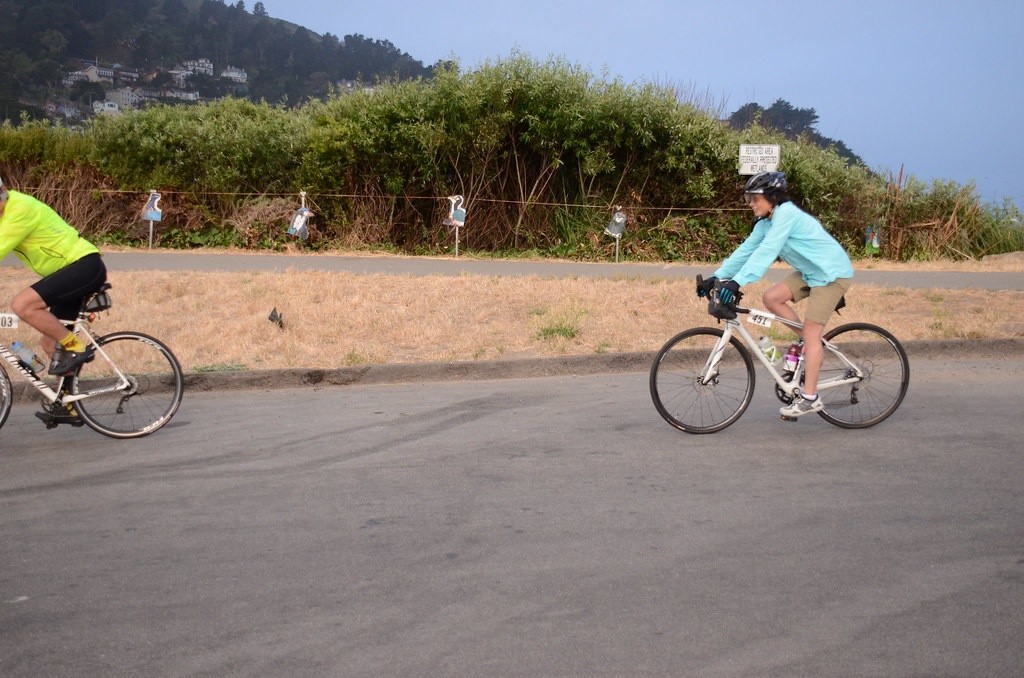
<box><xmin>782</xmin><ymin>341</ymin><xmax>799</xmax><ymax>372</ymax></box>
<box><xmin>12</xmin><ymin>341</ymin><xmax>45</xmax><ymax>374</ymax></box>
<box><xmin>759</xmin><ymin>336</ymin><xmax>783</xmax><ymax>367</ymax></box>
<box><xmin>49</xmin><ymin>342</ymin><xmax>65</xmax><ymax>370</ymax></box>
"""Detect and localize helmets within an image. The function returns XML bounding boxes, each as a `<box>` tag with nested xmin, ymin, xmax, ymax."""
<box><xmin>744</xmin><ymin>171</ymin><xmax>788</xmax><ymax>220</ymax></box>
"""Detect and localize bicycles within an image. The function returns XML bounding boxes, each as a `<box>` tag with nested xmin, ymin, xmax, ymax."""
<box><xmin>1</xmin><ymin>283</ymin><xmax>186</xmax><ymax>440</ymax></box>
<box><xmin>648</xmin><ymin>275</ymin><xmax>910</xmax><ymax>435</ymax></box>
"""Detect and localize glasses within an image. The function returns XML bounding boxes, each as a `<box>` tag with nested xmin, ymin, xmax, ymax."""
<box><xmin>749</xmin><ymin>196</ymin><xmax>766</xmax><ymax>202</ymax></box>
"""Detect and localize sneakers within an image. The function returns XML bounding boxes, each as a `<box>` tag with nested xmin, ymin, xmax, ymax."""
<box><xmin>47</xmin><ymin>344</ymin><xmax>96</xmax><ymax>375</ymax></box>
<box><xmin>33</xmin><ymin>406</ymin><xmax>84</xmax><ymax>427</ymax></box>
<box><xmin>780</xmin><ymin>395</ymin><xmax>825</xmax><ymax>417</ymax></box>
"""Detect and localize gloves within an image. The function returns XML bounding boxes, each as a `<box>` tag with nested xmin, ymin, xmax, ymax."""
<box><xmin>696</xmin><ymin>276</ymin><xmax>717</xmax><ymax>298</ymax></box>
<box><xmin>717</xmin><ymin>280</ymin><xmax>740</xmax><ymax>306</ymax></box>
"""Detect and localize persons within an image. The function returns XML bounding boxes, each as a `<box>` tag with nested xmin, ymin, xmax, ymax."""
<box><xmin>0</xmin><ymin>176</ymin><xmax>108</xmax><ymax>427</ymax></box>
<box><xmin>697</xmin><ymin>171</ymin><xmax>853</xmax><ymax>416</ymax></box>
